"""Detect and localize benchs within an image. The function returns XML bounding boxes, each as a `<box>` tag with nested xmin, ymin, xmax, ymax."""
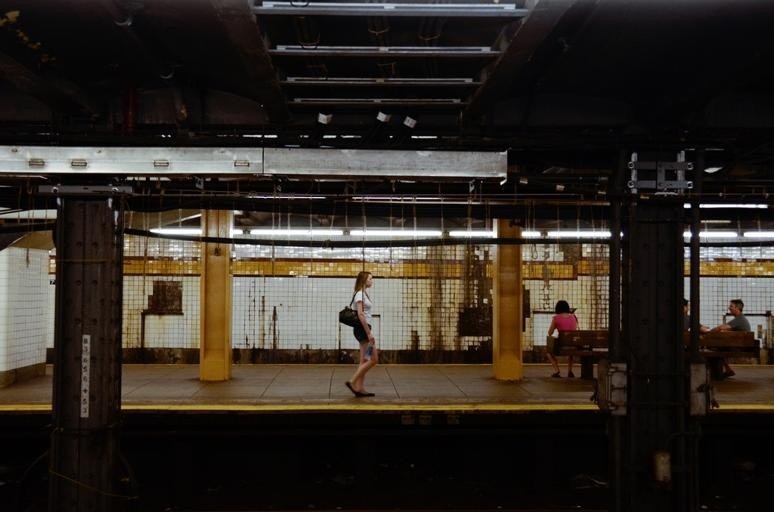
<box><xmin>546</xmin><ymin>328</ymin><xmax>760</xmax><ymax>381</ymax></box>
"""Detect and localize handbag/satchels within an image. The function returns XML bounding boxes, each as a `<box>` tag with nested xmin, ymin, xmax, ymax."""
<box><xmin>337</xmin><ymin>305</ymin><xmax>359</xmax><ymax>328</ymax></box>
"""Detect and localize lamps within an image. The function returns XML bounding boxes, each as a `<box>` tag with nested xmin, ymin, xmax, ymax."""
<box><xmin>349</xmin><ymin>192</ymin><xmax>443</xmax><ymax>236</ymax></box>
<box><xmin>450</xmin><ymin>198</ymin><xmax>540</xmax><ymax>238</ymax></box>
<box><xmin>149</xmin><ymin>183</ymin><xmax>246</xmax><ymax>237</ymax></box>
<box><xmin>249</xmin><ymin>184</ymin><xmax>344</xmax><ymax>237</ymax></box>
<box><xmin>683</xmin><ymin>197</ymin><xmax>774</xmax><ymax>239</ymax></box>
<box><xmin>546</xmin><ymin>198</ymin><xmax>625</xmax><ymax>238</ymax></box>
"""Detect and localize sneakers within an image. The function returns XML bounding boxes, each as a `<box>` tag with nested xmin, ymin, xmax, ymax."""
<box><xmin>711</xmin><ymin>369</ymin><xmax>736</xmax><ymax>379</ymax></box>
<box><xmin>344</xmin><ymin>381</ymin><xmax>375</xmax><ymax>397</ymax></box>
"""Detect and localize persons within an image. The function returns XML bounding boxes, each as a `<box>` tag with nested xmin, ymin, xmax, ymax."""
<box><xmin>546</xmin><ymin>298</ymin><xmax>577</xmax><ymax>378</ymax></box>
<box><xmin>344</xmin><ymin>270</ymin><xmax>379</xmax><ymax>398</ymax></box>
<box><xmin>683</xmin><ymin>297</ymin><xmax>711</xmax><ymax>350</ymax></box>
<box><xmin>705</xmin><ymin>299</ymin><xmax>752</xmax><ymax>382</ymax></box>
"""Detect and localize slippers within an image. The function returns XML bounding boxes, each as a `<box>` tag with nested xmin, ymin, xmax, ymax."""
<box><xmin>550</xmin><ymin>369</ymin><xmax>575</xmax><ymax>378</ymax></box>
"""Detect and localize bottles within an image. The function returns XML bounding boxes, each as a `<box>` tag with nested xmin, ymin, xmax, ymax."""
<box><xmin>364</xmin><ymin>342</ymin><xmax>374</xmax><ymax>361</ymax></box>
